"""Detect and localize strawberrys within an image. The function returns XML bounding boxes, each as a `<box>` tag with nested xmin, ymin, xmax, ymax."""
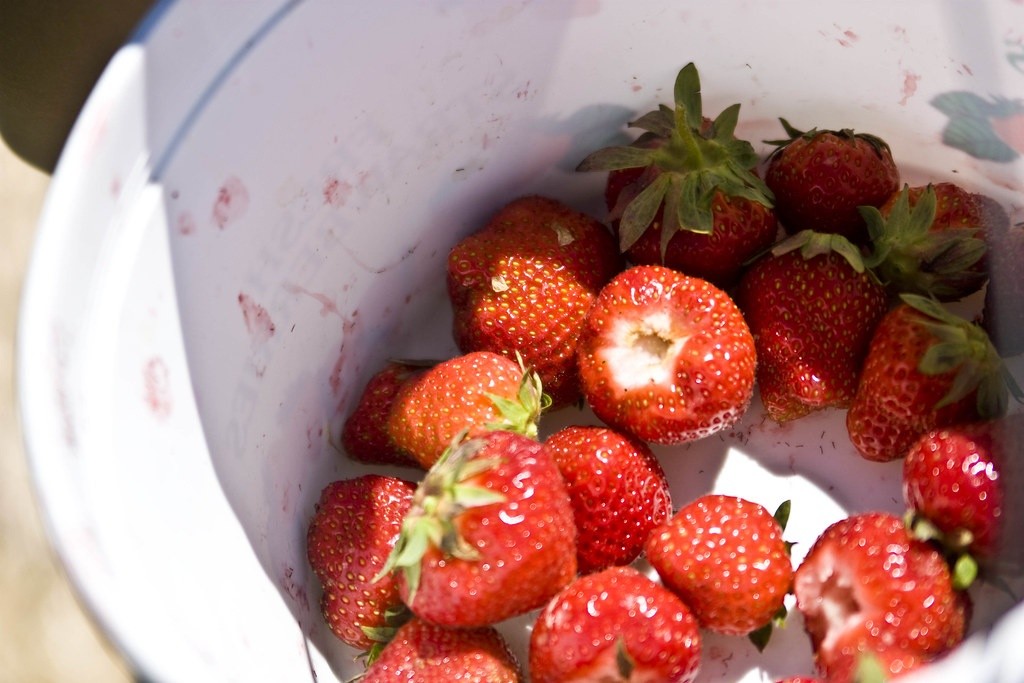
<box><xmin>303</xmin><ymin>58</ymin><xmax>1024</xmax><ymax>683</ymax></box>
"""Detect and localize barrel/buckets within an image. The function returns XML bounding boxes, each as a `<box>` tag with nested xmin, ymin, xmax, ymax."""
<box><xmin>15</xmin><ymin>0</ymin><xmax>1024</xmax><ymax>683</ymax></box>
